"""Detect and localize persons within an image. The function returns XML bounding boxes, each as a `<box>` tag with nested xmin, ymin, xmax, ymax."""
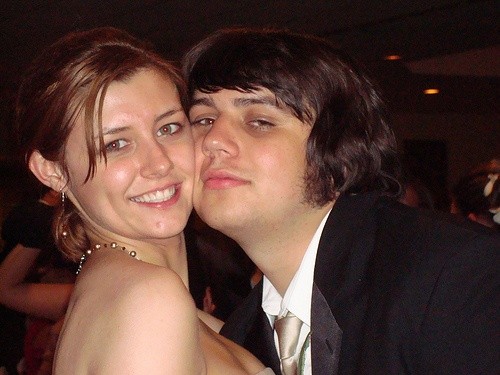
<box><xmin>0</xmin><ymin>190</ymin><xmax>264</xmax><ymax>375</ymax></box>
<box><xmin>180</xmin><ymin>26</ymin><xmax>500</xmax><ymax>375</ymax></box>
<box><xmin>399</xmin><ymin>148</ymin><xmax>500</xmax><ymax>231</ymax></box>
<box><xmin>17</xmin><ymin>28</ymin><xmax>276</xmax><ymax>375</ymax></box>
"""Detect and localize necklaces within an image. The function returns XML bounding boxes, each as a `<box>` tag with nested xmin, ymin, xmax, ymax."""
<box><xmin>75</xmin><ymin>243</ymin><xmax>137</xmax><ymax>274</ymax></box>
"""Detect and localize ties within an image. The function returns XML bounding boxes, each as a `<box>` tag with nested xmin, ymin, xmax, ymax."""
<box><xmin>274</xmin><ymin>317</ymin><xmax>304</xmax><ymax>375</ymax></box>
